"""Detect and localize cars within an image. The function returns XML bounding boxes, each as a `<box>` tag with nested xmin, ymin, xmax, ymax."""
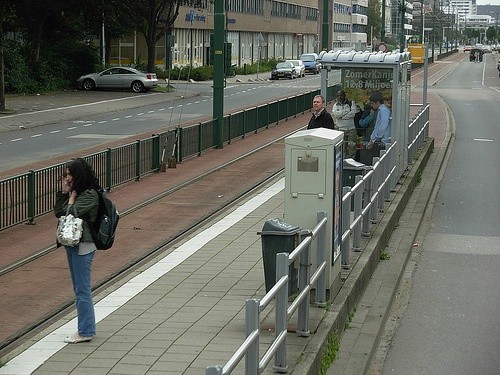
<box><xmin>271</xmin><ymin>62</ymin><xmax>298</xmax><ymax>80</ymax></box>
<box><xmin>464</xmin><ymin>45</ymin><xmax>473</xmax><ymax>52</ymax></box>
<box><xmin>469</xmin><ymin>46</ymin><xmax>500</xmax><ymax>62</ymax></box>
<box><xmin>285</xmin><ymin>60</ymin><xmax>306</xmax><ymax>78</ymax></box>
<box><xmin>77</xmin><ymin>67</ymin><xmax>159</xmax><ymax>92</ymax></box>
<box><xmin>300</xmin><ymin>53</ymin><xmax>322</xmax><ymax>75</ymax></box>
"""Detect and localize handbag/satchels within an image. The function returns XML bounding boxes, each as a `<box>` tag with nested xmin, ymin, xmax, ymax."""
<box><xmin>55</xmin><ymin>202</ymin><xmax>82</xmax><ymax>247</ymax></box>
<box><xmin>355</xmin><ymin>145</ymin><xmax>372</xmax><ymax>165</ymax></box>
<box><xmin>371</xmin><ymin>141</ymin><xmax>385</xmax><ymax>157</ymax></box>
<box><xmin>355</xmin><ymin>111</ymin><xmax>365</xmax><ymax>136</ymax></box>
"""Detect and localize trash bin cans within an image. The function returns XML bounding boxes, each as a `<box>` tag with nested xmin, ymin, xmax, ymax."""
<box><xmin>257</xmin><ymin>217</ymin><xmax>303</xmax><ymax>299</ymax></box>
<box><xmin>343</xmin><ymin>158</ymin><xmax>367</xmax><ymax>210</ymax></box>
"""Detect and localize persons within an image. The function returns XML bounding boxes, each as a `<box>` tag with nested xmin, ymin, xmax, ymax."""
<box><xmin>474</xmin><ymin>50</ymin><xmax>480</xmax><ymax>63</ymax></box>
<box><xmin>308</xmin><ymin>95</ymin><xmax>334</xmax><ymax>130</ymax></box>
<box><xmin>358</xmin><ymin>102</ymin><xmax>381</xmax><ymax>166</ymax></box>
<box><xmin>332</xmin><ymin>89</ymin><xmax>358</xmax><ymax>160</ymax></box>
<box><xmin>367</xmin><ymin>93</ymin><xmax>391</xmax><ymax>150</ymax></box>
<box><xmin>54</xmin><ymin>158</ymin><xmax>103</xmax><ymax>343</ymax></box>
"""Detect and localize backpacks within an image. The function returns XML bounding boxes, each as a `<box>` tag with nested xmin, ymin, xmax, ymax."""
<box><xmin>81</xmin><ymin>188</ymin><xmax>119</xmax><ymax>250</ymax></box>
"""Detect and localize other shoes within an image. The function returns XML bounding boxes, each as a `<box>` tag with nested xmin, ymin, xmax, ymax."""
<box><xmin>64</xmin><ymin>331</ymin><xmax>93</xmax><ymax>343</ymax></box>
